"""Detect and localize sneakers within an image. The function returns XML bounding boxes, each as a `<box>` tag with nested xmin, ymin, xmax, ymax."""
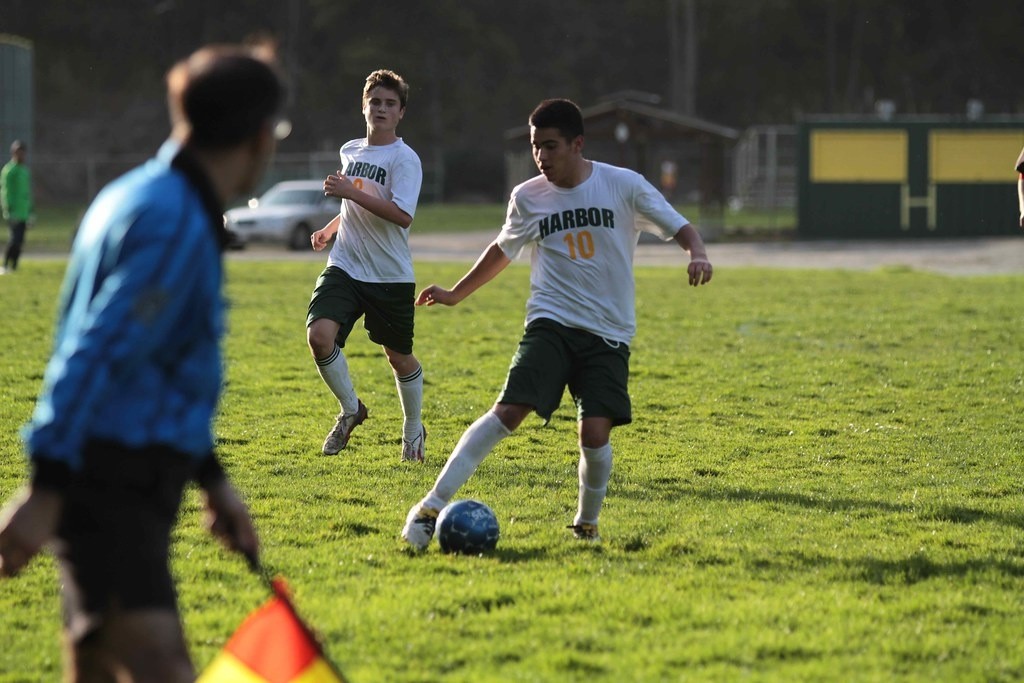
<box><xmin>401</xmin><ymin>424</ymin><xmax>427</xmax><ymax>463</ymax></box>
<box><xmin>402</xmin><ymin>500</ymin><xmax>439</xmax><ymax>551</ymax></box>
<box><xmin>322</xmin><ymin>398</ymin><xmax>368</xmax><ymax>454</ymax></box>
<box><xmin>567</xmin><ymin>521</ymin><xmax>600</xmax><ymax>543</ymax></box>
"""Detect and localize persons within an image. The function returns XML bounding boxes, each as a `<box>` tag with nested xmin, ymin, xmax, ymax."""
<box><xmin>305</xmin><ymin>69</ymin><xmax>427</xmax><ymax>464</ymax></box>
<box><xmin>1</xmin><ymin>139</ymin><xmax>32</xmax><ymax>276</ymax></box>
<box><xmin>0</xmin><ymin>48</ymin><xmax>293</xmax><ymax>683</ymax></box>
<box><xmin>402</xmin><ymin>98</ymin><xmax>714</xmax><ymax>556</ymax></box>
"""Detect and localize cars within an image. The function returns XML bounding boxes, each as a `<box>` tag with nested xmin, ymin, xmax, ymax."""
<box><xmin>223</xmin><ymin>181</ymin><xmax>342</xmax><ymax>251</ymax></box>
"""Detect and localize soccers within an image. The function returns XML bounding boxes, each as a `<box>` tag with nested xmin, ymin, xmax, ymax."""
<box><xmin>434</xmin><ymin>498</ymin><xmax>500</xmax><ymax>554</ymax></box>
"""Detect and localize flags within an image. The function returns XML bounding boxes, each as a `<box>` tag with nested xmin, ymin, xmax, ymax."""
<box><xmin>189</xmin><ymin>582</ymin><xmax>338</xmax><ymax>682</ymax></box>
